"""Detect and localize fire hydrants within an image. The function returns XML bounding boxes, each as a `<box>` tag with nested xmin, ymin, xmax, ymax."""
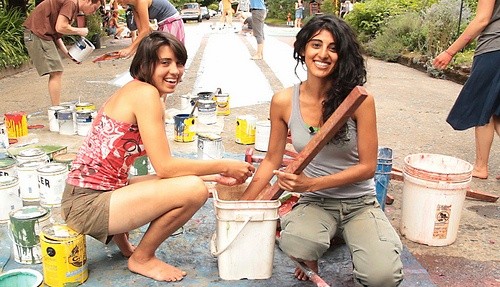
<box><xmin>287</xmin><ymin>13</ymin><xmax>292</xmax><ymax>25</ymax></box>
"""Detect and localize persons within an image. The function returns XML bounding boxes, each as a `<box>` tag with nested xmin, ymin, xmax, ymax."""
<box><xmin>22</xmin><ymin>0</ymin><xmax>304</xmax><ymax>106</ymax></box>
<box><xmin>60</xmin><ymin>32</ymin><xmax>254</xmax><ymax>282</ymax></box>
<box><xmin>433</xmin><ymin>0</ymin><xmax>500</xmax><ymax>179</ymax></box>
<box><xmin>240</xmin><ymin>15</ymin><xmax>405</xmax><ymax>287</ymax></box>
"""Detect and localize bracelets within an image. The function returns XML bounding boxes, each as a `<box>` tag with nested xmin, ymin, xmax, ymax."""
<box><xmin>444</xmin><ymin>51</ymin><xmax>453</xmax><ymax>57</ymax></box>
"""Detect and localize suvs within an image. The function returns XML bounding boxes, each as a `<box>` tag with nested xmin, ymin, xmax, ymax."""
<box><xmin>180</xmin><ymin>2</ymin><xmax>202</xmax><ymax>23</ymax></box>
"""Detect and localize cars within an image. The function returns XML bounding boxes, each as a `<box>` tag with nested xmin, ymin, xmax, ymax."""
<box><xmin>200</xmin><ymin>7</ymin><xmax>210</xmax><ymax>20</ymax></box>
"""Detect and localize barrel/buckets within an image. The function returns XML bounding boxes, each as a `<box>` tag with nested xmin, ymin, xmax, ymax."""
<box><xmin>68</xmin><ymin>37</ymin><xmax>95</xmax><ymax>65</ymax></box>
<box><xmin>8</xmin><ymin>206</ymin><xmax>51</xmax><ymax>265</ymax></box>
<box><xmin>197</xmin><ymin>132</ymin><xmax>225</xmax><ymax>161</ymax></box>
<box><xmin>48</xmin><ymin>98</ymin><xmax>97</xmax><ymax>136</ymax></box>
<box><xmin>173</xmin><ymin>113</ymin><xmax>196</xmax><ymax>143</ymax></box>
<box><xmin>129</xmin><ymin>156</ymin><xmax>148</xmax><ymax>176</ymax></box>
<box><xmin>235</xmin><ymin>115</ymin><xmax>258</xmax><ymax>145</ymax></box>
<box><xmin>190</xmin><ymin>90</ymin><xmax>231</xmax><ymax>125</ymax></box>
<box><xmin>0</xmin><ymin>121</ymin><xmax>72</xmax><ymax>224</ymax></box>
<box><xmin>4</xmin><ymin>111</ymin><xmax>28</xmax><ymax>138</ymax></box>
<box><xmin>39</xmin><ymin>222</ymin><xmax>88</xmax><ymax>287</ymax></box>
<box><xmin>0</xmin><ymin>268</ymin><xmax>44</xmax><ymax>287</ymax></box>
<box><xmin>374</xmin><ymin>147</ymin><xmax>394</xmax><ymax>211</ymax></box>
<box><xmin>210</xmin><ymin>184</ymin><xmax>281</xmax><ymax>280</ymax></box>
<box><xmin>399</xmin><ymin>153</ymin><xmax>474</xmax><ymax>247</ymax></box>
<box><xmin>254</xmin><ymin>120</ymin><xmax>270</xmax><ymax>152</ymax></box>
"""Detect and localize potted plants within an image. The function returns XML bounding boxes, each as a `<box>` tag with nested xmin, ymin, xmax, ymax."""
<box><xmin>85</xmin><ymin>14</ymin><xmax>102</xmax><ymax>48</ymax></box>
